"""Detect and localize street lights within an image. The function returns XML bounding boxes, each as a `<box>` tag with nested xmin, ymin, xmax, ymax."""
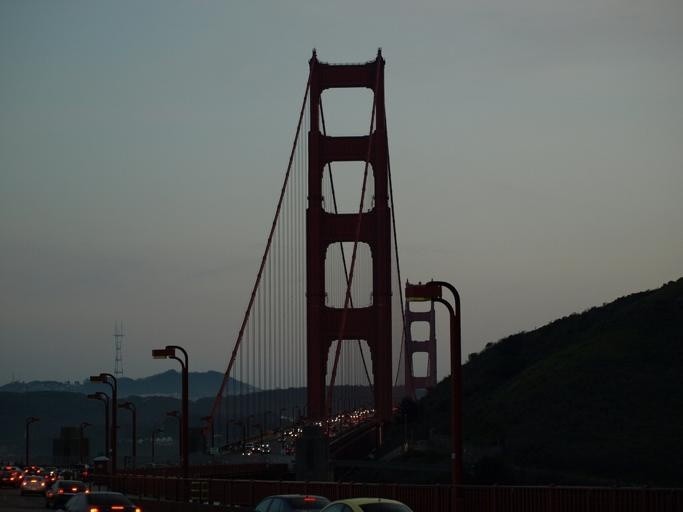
<box><xmin>84</xmin><ymin>371</ymin><xmax>137</xmax><ymax>471</ymax></box>
<box><xmin>26</xmin><ymin>416</ymin><xmax>40</xmax><ymax>465</ymax></box>
<box><xmin>403</xmin><ymin>279</ymin><xmax>461</xmax><ymax>512</ymax></box>
<box><xmin>151</xmin><ymin>346</ymin><xmax>189</xmax><ymax>471</ymax></box>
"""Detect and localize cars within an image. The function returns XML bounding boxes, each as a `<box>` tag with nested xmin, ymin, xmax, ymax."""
<box><xmin>254</xmin><ymin>494</ymin><xmax>413</xmax><ymax>512</ymax></box>
<box><xmin>1</xmin><ymin>466</ymin><xmax>143</xmax><ymax>512</ymax></box>
